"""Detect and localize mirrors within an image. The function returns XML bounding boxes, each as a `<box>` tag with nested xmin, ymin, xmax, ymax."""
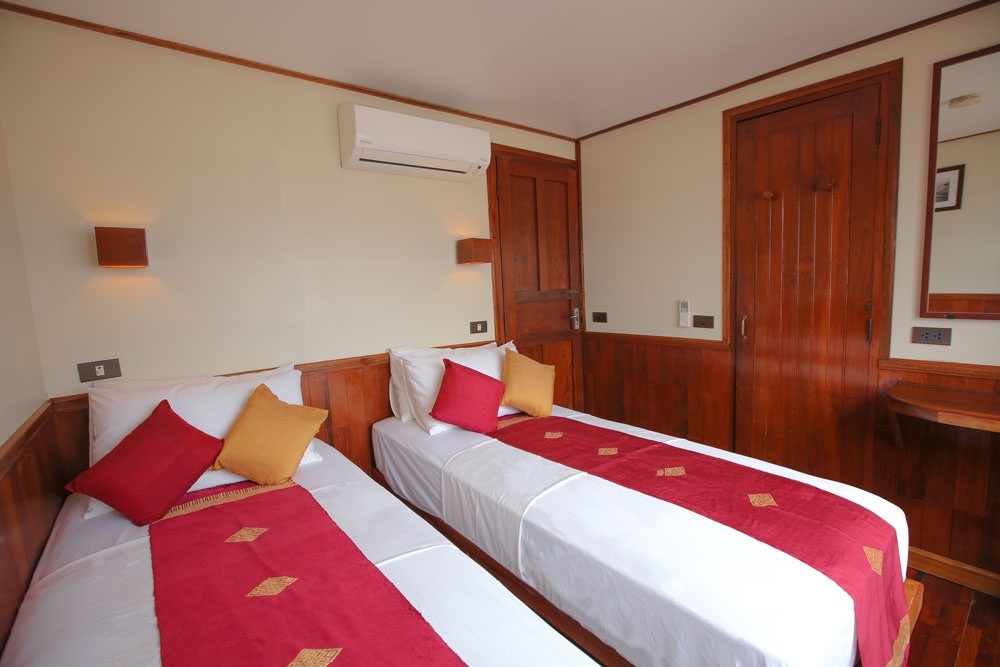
<box><xmin>920</xmin><ymin>42</ymin><xmax>1000</xmax><ymax>321</ymax></box>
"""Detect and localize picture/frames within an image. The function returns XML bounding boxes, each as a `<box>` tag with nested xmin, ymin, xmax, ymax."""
<box><xmin>934</xmin><ymin>164</ymin><xmax>966</xmax><ymax>212</ymax></box>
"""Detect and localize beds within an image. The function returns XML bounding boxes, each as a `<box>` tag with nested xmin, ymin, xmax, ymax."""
<box><xmin>370</xmin><ymin>401</ymin><xmax>910</xmax><ymax>667</ymax></box>
<box><xmin>0</xmin><ymin>437</ymin><xmax>606</xmax><ymax>667</ymax></box>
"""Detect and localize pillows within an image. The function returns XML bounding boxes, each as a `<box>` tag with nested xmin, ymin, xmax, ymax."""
<box><xmin>64</xmin><ymin>399</ymin><xmax>226</xmax><ymax>527</ymax></box>
<box><xmin>206</xmin><ymin>384</ymin><xmax>329</xmax><ymax>486</ymax></box>
<box><xmin>399</xmin><ymin>340</ymin><xmax>523</xmax><ymax>436</ymax></box>
<box><xmin>499</xmin><ymin>348</ymin><xmax>556</xmax><ymax>418</ymax></box>
<box><xmin>386</xmin><ymin>342</ymin><xmax>498</xmax><ymax>424</ymax></box>
<box><xmin>82</xmin><ymin>364</ymin><xmax>294</xmax><ymax>468</ymax></box>
<box><xmin>428</xmin><ymin>359</ymin><xmax>505</xmax><ymax>435</ymax></box>
<box><xmin>82</xmin><ymin>370</ymin><xmax>304</xmax><ymax>522</ymax></box>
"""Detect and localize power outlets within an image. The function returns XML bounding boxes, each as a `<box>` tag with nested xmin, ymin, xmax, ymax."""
<box><xmin>77</xmin><ymin>357</ymin><xmax>123</xmax><ymax>384</ymax></box>
<box><xmin>592</xmin><ymin>312</ymin><xmax>607</xmax><ymax>323</ymax></box>
<box><xmin>470</xmin><ymin>321</ymin><xmax>487</xmax><ymax>334</ymax></box>
<box><xmin>912</xmin><ymin>327</ymin><xmax>952</xmax><ymax>346</ymax></box>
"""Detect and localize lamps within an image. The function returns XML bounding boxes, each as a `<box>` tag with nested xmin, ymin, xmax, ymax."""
<box><xmin>94</xmin><ymin>227</ymin><xmax>149</xmax><ymax>268</ymax></box>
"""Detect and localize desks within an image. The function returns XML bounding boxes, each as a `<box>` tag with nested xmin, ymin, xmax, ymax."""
<box><xmin>885</xmin><ymin>380</ymin><xmax>1000</xmax><ymax>451</ymax></box>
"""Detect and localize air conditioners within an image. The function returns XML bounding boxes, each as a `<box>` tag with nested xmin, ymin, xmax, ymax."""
<box><xmin>338</xmin><ymin>102</ymin><xmax>490</xmax><ymax>185</ymax></box>
